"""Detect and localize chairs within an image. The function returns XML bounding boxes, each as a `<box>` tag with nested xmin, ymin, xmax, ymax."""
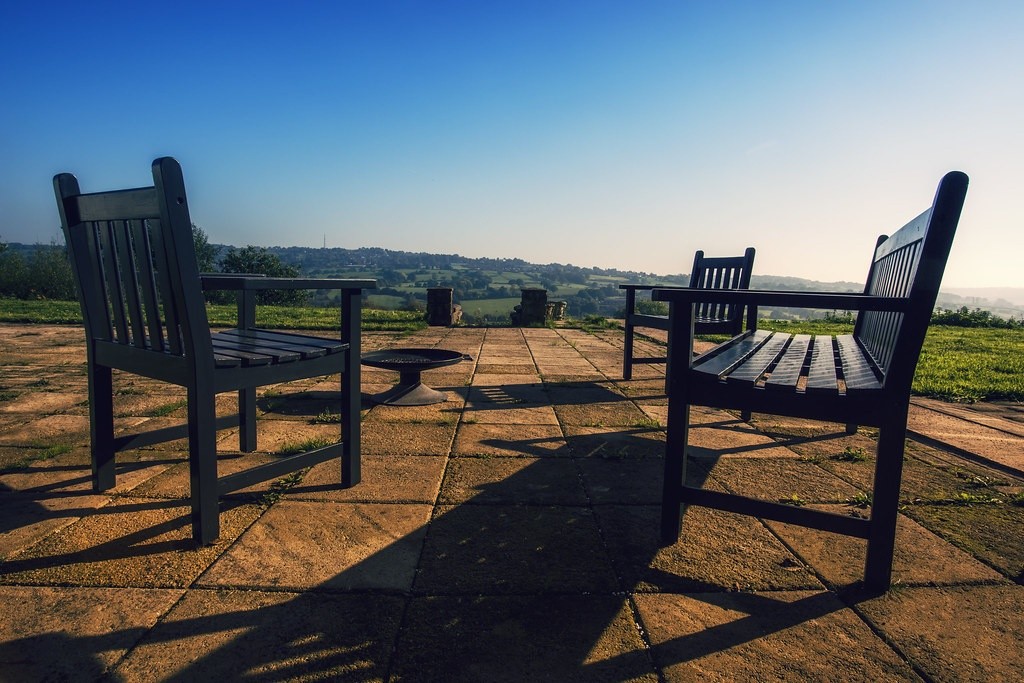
<box><xmin>619</xmin><ymin>247</ymin><xmax>758</xmax><ymax>381</ymax></box>
<box><xmin>53</xmin><ymin>156</ymin><xmax>378</xmax><ymax>549</ymax></box>
<box><xmin>662</xmin><ymin>171</ymin><xmax>970</xmax><ymax>596</ymax></box>
